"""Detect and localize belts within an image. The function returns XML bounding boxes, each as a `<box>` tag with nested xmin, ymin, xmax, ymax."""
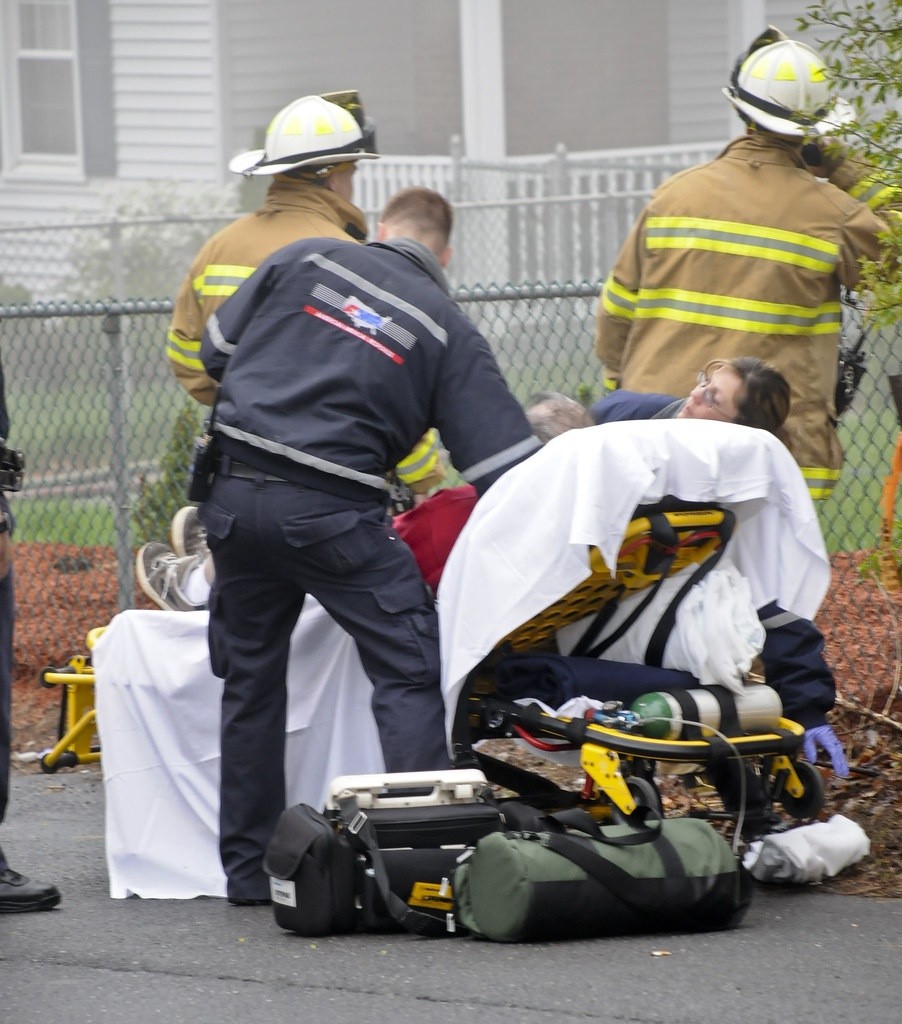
<box><xmin>216</xmin><ymin>459</ymin><xmax>290</xmax><ymax>482</ymax></box>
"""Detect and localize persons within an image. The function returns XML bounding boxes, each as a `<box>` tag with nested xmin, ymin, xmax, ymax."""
<box><xmin>594</xmin><ymin>26</ymin><xmax>901</xmax><ymax>516</ymax></box>
<box><xmin>135</xmin><ymin>391</ymin><xmax>602</xmax><ymax>611</ymax></box>
<box><xmin>587</xmin><ymin>357</ymin><xmax>852</xmax><ymax>774</ymax></box>
<box><xmin>165</xmin><ymin>93</ymin><xmax>450</xmax><ymax>520</ymax></box>
<box><xmin>187</xmin><ymin>189</ymin><xmax>547</xmax><ymax>903</ymax></box>
<box><xmin>0</xmin><ymin>352</ymin><xmax>65</xmax><ymax>913</ymax></box>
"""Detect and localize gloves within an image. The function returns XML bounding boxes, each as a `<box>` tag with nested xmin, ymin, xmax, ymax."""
<box><xmin>802</xmin><ymin>725</ymin><xmax>849</xmax><ymax>776</ymax></box>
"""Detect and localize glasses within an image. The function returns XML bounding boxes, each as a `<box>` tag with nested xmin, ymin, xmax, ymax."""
<box><xmin>697</xmin><ymin>370</ymin><xmax>734</xmax><ymax>422</ymax></box>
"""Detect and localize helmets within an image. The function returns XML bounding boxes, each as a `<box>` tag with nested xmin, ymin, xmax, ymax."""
<box><xmin>228</xmin><ymin>90</ymin><xmax>381</xmax><ymax>176</ymax></box>
<box><xmin>721</xmin><ymin>24</ymin><xmax>857</xmax><ymax>136</ymax></box>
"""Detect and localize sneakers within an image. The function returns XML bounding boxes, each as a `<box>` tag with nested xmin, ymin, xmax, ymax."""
<box><xmin>136</xmin><ymin>542</ymin><xmax>209</xmax><ymax>611</ymax></box>
<box><xmin>0</xmin><ymin>869</ymin><xmax>61</xmax><ymax>912</ymax></box>
<box><xmin>171</xmin><ymin>505</ymin><xmax>211</xmax><ymax>558</ymax></box>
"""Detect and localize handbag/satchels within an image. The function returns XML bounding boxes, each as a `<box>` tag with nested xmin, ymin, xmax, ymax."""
<box><xmin>261</xmin><ymin>803</ymin><xmax>550</xmax><ymax>939</ymax></box>
<box><xmin>454</xmin><ymin>805</ymin><xmax>741</xmax><ymax>942</ymax></box>
